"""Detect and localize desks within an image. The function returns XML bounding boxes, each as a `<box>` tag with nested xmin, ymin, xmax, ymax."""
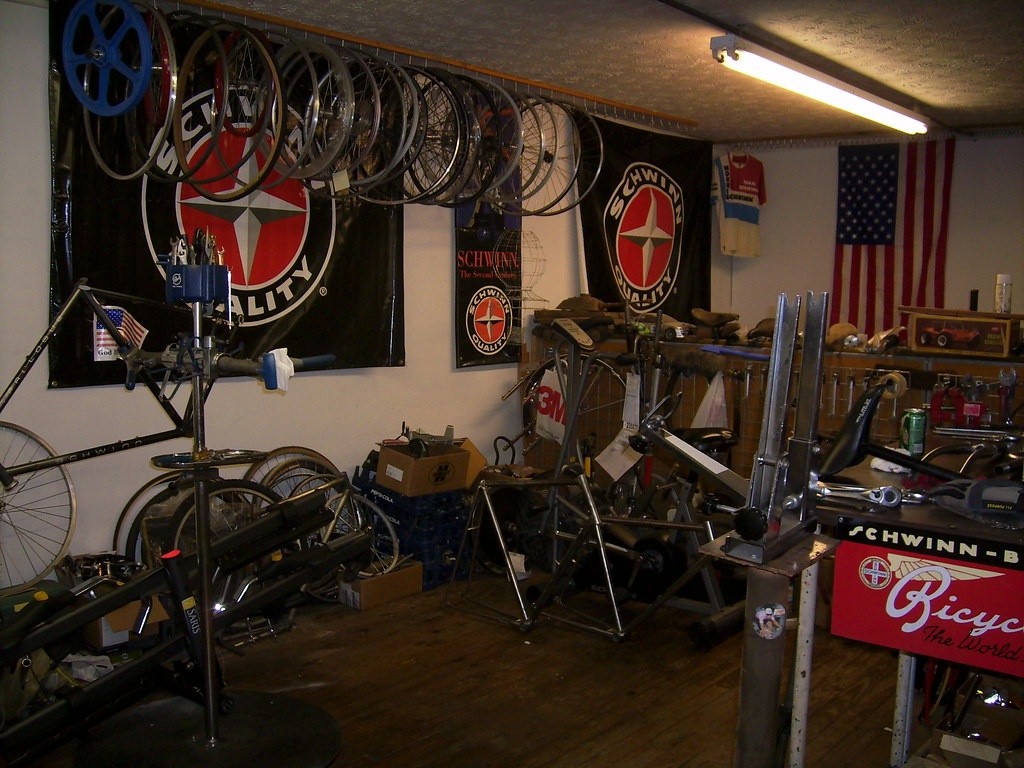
<box><xmin>787</xmin><ymin>439</ymin><xmax>1024</xmax><ymax>768</ymax></box>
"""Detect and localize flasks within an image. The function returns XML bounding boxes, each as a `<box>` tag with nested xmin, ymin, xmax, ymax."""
<box><xmin>994</xmin><ymin>274</ymin><xmax>1013</xmax><ymax>314</ymax></box>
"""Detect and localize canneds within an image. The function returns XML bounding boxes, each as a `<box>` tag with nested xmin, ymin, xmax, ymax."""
<box><xmin>899</xmin><ymin>408</ymin><xmax>928</xmax><ymax>458</ymax></box>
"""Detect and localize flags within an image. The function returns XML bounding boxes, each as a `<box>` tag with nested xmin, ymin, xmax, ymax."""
<box><xmin>828</xmin><ymin>140</ymin><xmax>956</xmax><ymax>342</ymax></box>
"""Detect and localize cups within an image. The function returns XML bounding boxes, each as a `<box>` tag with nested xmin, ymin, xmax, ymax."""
<box><xmin>971</xmin><ymin>289</ymin><xmax>979</xmax><ymax>311</ymax></box>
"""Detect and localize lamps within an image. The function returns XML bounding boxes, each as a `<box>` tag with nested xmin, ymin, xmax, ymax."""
<box><xmin>709</xmin><ymin>34</ymin><xmax>931</xmax><ymax>136</ymax></box>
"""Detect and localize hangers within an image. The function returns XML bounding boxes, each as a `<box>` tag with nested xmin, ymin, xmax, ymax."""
<box><xmin>731</xmin><ymin>144</ymin><xmax>745</xmax><ymax>153</ymax></box>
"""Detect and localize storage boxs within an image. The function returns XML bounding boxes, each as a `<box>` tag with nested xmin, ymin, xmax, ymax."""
<box><xmin>375</xmin><ymin>442</ymin><xmax>471</xmax><ymax>497</ymax></box>
<box><xmin>363</xmin><ymin>479</ymin><xmax>468</xmax><ymax>590</ymax></box>
<box><xmin>995</xmin><ymin>274</ymin><xmax>1013</xmax><ymax>314</ymax></box>
<box><xmin>459</xmin><ymin>437</ymin><xmax>488</xmax><ymax>490</ymax></box>
<box><xmin>83</xmin><ymin>595</ymin><xmax>170</xmax><ymax>649</ymax></box>
<box><xmin>338</xmin><ymin>555</ymin><xmax>423</xmax><ymax>611</ymax></box>
